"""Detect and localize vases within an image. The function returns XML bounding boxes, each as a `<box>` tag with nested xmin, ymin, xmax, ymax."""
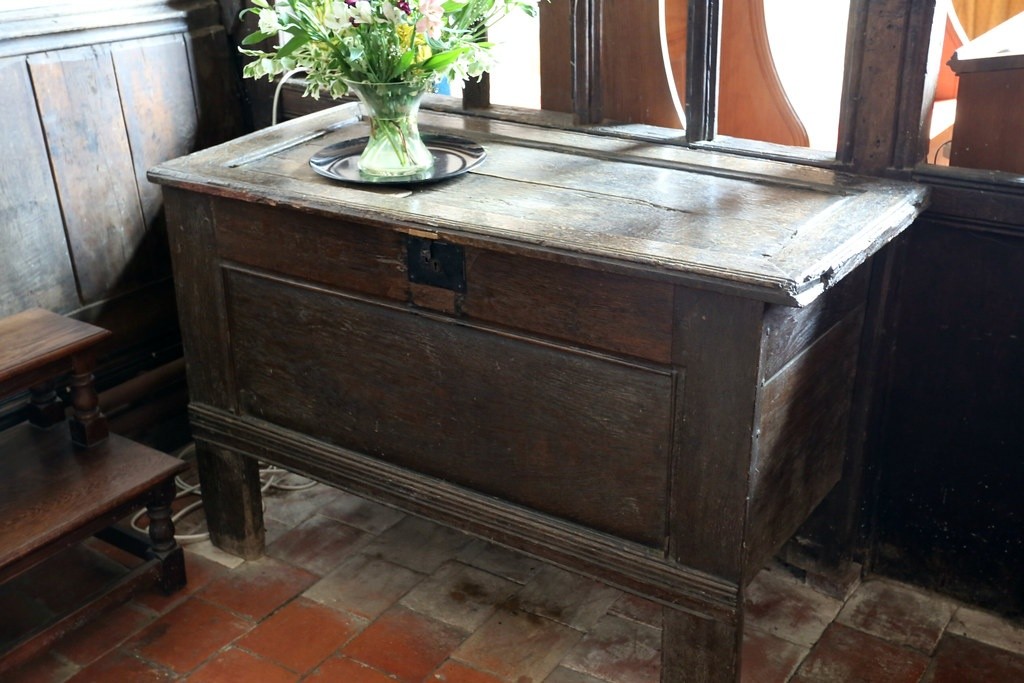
<box><xmin>339</xmin><ymin>76</ymin><xmax>435</xmax><ymax>176</ymax></box>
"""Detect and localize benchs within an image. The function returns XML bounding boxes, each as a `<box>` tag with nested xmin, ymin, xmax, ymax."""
<box><xmin>0</xmin><ymin>306</ymin><xmax>115</xmax><ymax>462</ymax></box>
<box><xmin>0</xmin><ymin>421</ymin><xmax>191</xmax><ymax>678</ymax></box>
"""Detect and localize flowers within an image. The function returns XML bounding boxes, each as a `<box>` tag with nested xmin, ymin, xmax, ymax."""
<box><xmin>236</xmin><ymin>0</ymin><xmax>556</xmax><ymax>172</ymax></box>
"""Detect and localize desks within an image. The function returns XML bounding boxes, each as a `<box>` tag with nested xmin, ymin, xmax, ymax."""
<box><xmin>143</xmin><ymin>100</ymin><xmax>934</xmax><ymax>683</ymax></box>
